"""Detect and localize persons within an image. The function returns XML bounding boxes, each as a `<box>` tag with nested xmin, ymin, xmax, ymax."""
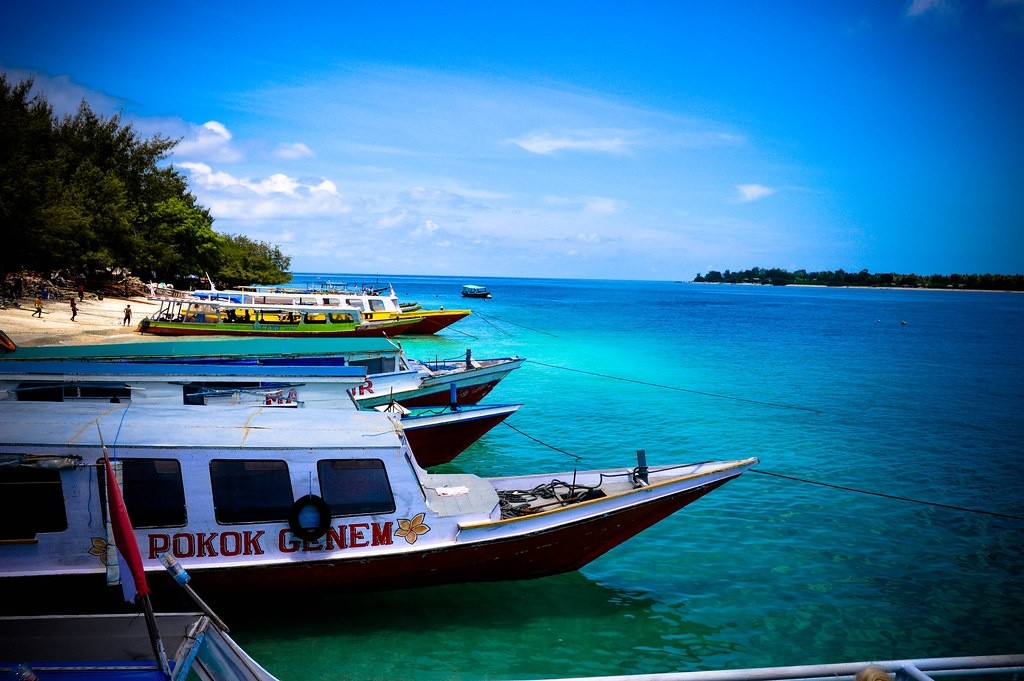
<box><xmin>223</xmin><ymin>309</ymin><xmax>250</xmax><ymax>324</ymax></box>
<box><xmin>70</xmin><ymin>298</ymin><xmax>79</xmax><ymax>321</ymax></box>
<box><xmin>78</xmin><ymin>282</ymin><xmax>86</xmax><ymax>302</ymax></box>
<box><xmin>123</xmin><ymin>304</ymin><xmax>132</xmax><ymax>327</ymax></box>
<box><xmin>287</xmin><ymin>312</ymin><xmax>294</xmax><ymax>325</ymax></box>
<box><xmin>31</xmin><ymin>296</ymin><xmax>43</xmax><ymax>319</ymax></box>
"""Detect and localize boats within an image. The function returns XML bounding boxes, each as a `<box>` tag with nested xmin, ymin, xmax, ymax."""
<box><xmin>0</xmin><ymin>337</ymin><xmax>525</xmax><ymax>469</ymax></box>
<box><xmin>181</xmin><ymin>282</ymin><xmax>471</xmax><ymax>335</ymax></box>
<box><xmin>0</xmin><ymin>614</ymin><xmax>280</xmax><ymax>681</ymax></box>
<box><xmin>133</xmin><ymin>294</ymin><xmax>426</xmax><ymax>337</ymax></box>
<box><xmin>460</xmin><ymin>284</ymin><xmax>492</xmax><ymax>299</ymax></box>
<box><xmin>2</xmin><ymin>399</ymin><xmax>762</xmax><ymax>613</ymax></box>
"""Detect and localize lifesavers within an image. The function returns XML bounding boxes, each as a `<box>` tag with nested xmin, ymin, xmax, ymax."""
<box><xmin>288</xmin><ymin>494</ymin><xmax>331</xmax><ymax>541</ymax></box>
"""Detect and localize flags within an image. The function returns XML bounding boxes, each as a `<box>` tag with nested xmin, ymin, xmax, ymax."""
<box><xmin>102</xmin><ymin>448</ymin><xmax>150</xmax><ymax>604</ymax></box>
